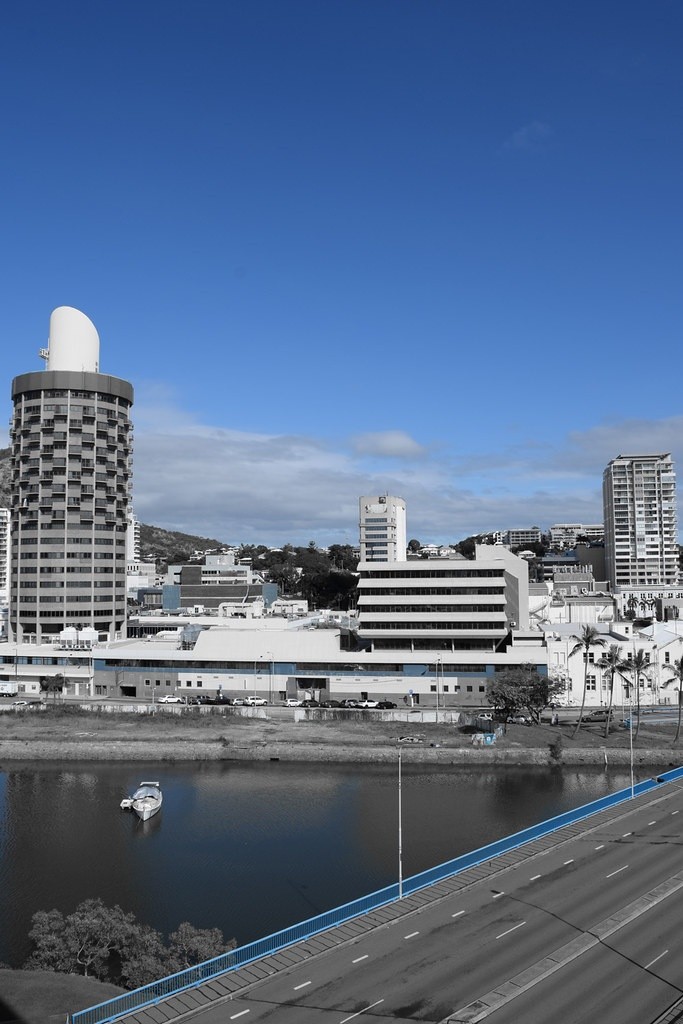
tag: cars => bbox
[478,713,493,721]
[180,695,231,705]
[340,698,359,708]
[157,695,182,704]
[26,700,44,706]
[229,698,244,706]
[301,699,322,708]
[507,714,532,725]
[12,700,27,705]
[377,701,397,709]
[283,699,302,708]
[322,699,340,708]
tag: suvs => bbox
[243,696,268,707]
[358,699,379,709]
[583,709,615,723]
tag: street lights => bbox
[437,652,445,707]
[254,656,263,707]
[436,659,440,712]
[64,653,73,688]
[267,651,275,704]
[13,648,18,680]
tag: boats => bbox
[119,781,163,821]
